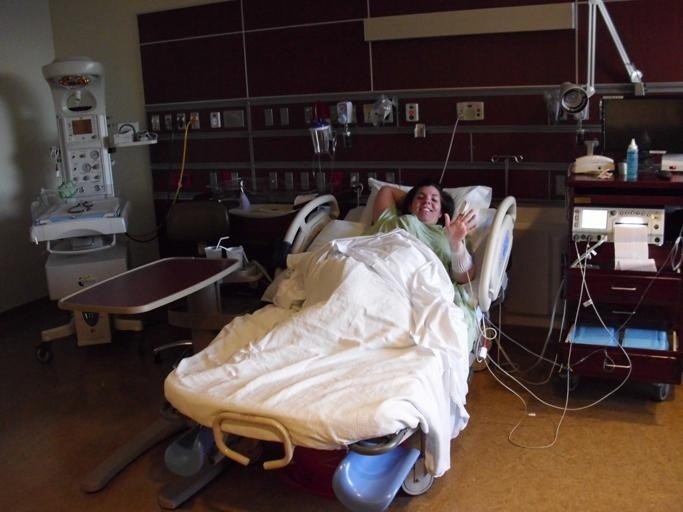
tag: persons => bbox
[358,182,478,285]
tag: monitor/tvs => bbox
[599,94,683,171]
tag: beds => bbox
[154,193,517,512]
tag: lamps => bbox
[557,1,645,113]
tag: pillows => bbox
[361,177,493,246]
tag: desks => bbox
[58,257,240,354]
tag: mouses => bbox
[656,170,672,180]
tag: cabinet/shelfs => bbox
[562,189,681,386]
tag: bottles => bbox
[626,137,639,181]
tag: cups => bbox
[310,117,329,152]
[204,246,223,259]
[226,247,243,270]
